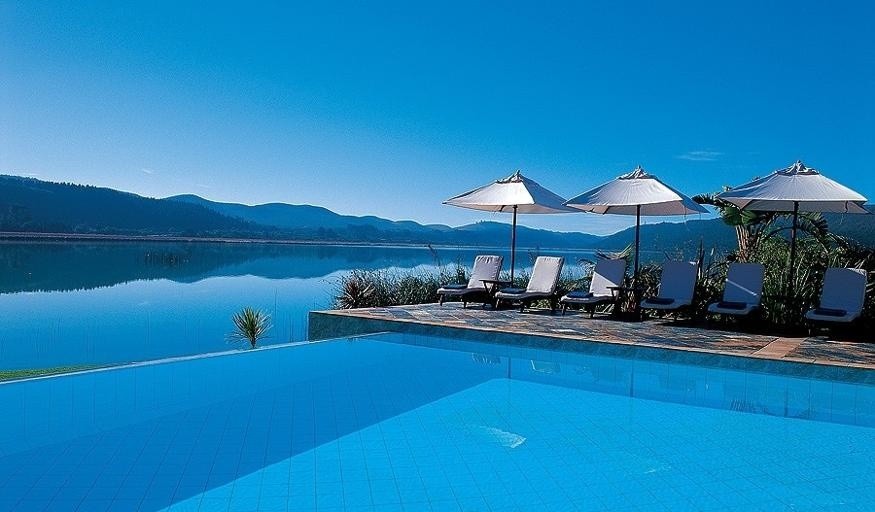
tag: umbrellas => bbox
[442,165,711,289]
[715,160,875,308]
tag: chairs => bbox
[470,352,501,368]
[803,267,868,336]
[639,261,698,324]
[436,255,506,308]
[559,258,628,319]
[494,255,565,314]
[531,359,560,376]
[703,262,766,329]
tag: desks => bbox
[608,286,644,320]
[479,279,512,309]
[767,295,805,335]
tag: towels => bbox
[500,287,527,294]
[566,290,594,298]
[646,296,675,305]
[814,307,847,317]
[717,301,747,310]
[443,284,467,289]
